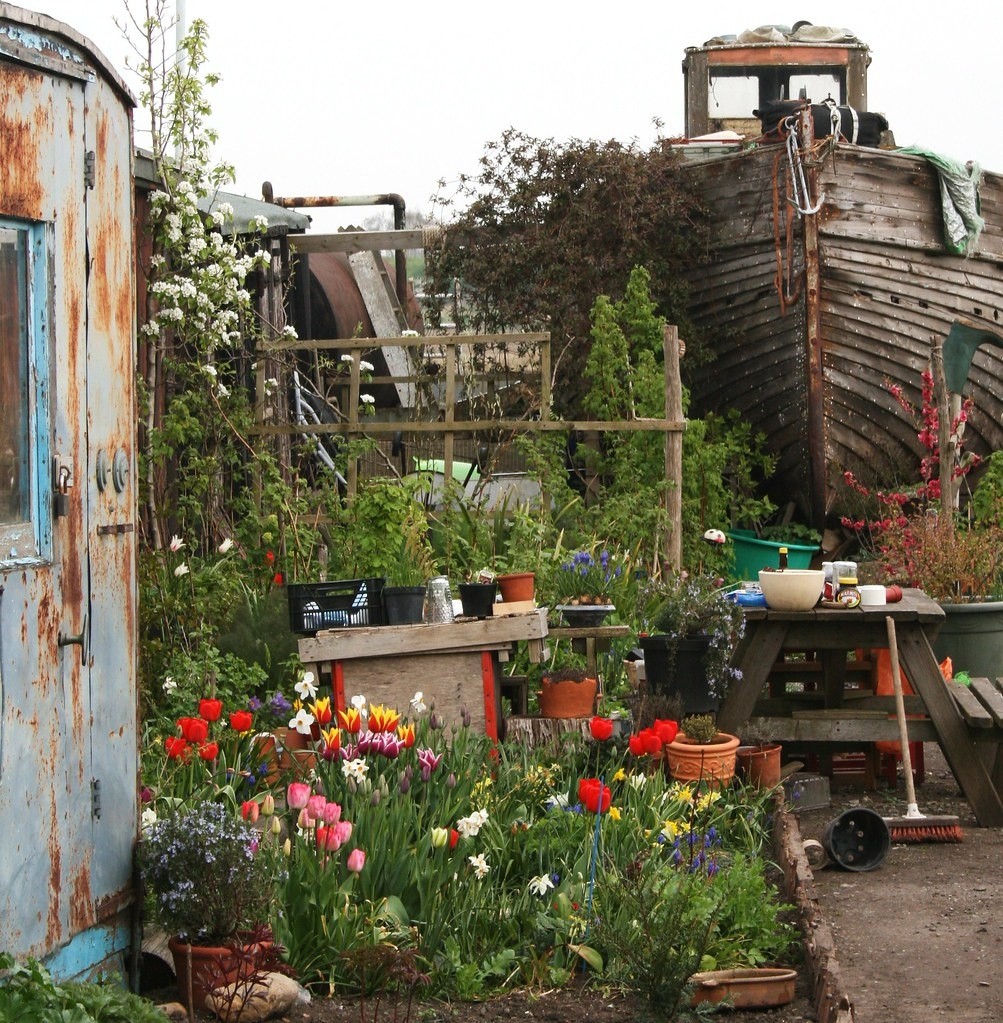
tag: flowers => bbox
[547,550,629,618]
[639,577,745,699]
[134,801,289,940]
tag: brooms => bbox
[879,616,968,844]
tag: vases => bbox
[757,569,826,611]
[168,933,274,1003]
[496,569,535,603]
[822,808,891,873]
[382,587,426,624]
[554,604,617,627]
[458,584,497,617]
[693,968,796,1002]
[639,632,716,701]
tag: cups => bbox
[861,584,886,606]
[822,561,857,602]
[424,574,455,625]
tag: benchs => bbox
[948,677,1003,727]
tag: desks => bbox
[718,589,1003,824]
[297,606,548,750]
[542,626,630,684]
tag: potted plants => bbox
[739,718,782,788]
[541,664,598,718]
[725,446,822,581]
[665,714,740,790]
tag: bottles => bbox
[779,547,790,570]
[834,578,862,608]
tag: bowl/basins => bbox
[759,569,825,611]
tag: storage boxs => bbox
[286,577,384,632]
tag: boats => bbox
[548,22,1003,562]
